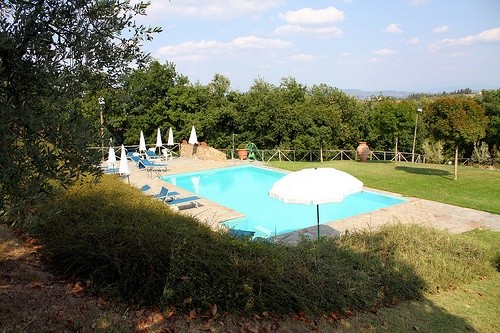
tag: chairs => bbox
[138,184,200,211]
[96,148,172,181]
[227,229,256,241]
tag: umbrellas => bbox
[139,131,146,153]
[109,138,117,168]
[157,128,162,155]
[191,176,200,193]
[168,127,174,146]
[171,178,176,185]
[119,144,130,182]
[269,168,363,239]
[189,126,198,144]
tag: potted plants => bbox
[236,144,250,160]
